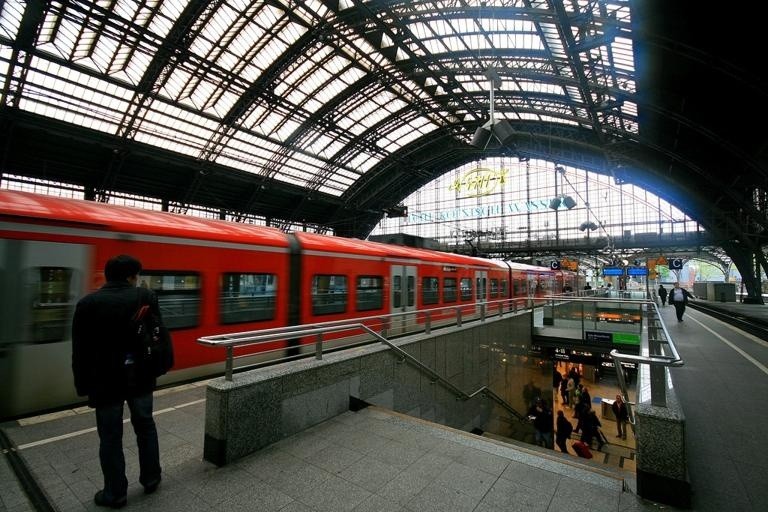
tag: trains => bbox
[0,190,576,420]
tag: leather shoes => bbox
[95,489,127,509]
[144,476,162,493]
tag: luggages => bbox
[572,442,592,459]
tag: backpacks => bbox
[125,286,174,378]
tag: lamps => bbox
[550,163,577,212]
[469,66,518,151]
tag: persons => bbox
[669,282,693,322]
[612,393,637,440]
[72,253,174,509]
[584,282,591,290]
[527,362,604,454]
[658,285,667,306]
[563,282,573,293]
